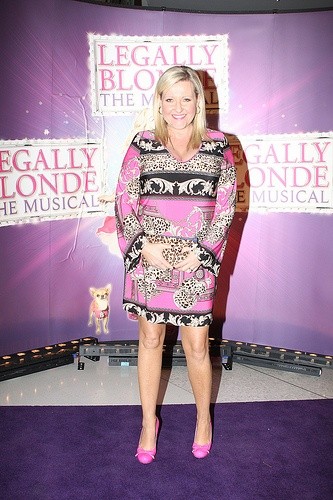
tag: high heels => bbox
[135,415,159,465]
[191,418,212,458]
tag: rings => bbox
[187,268,191,273]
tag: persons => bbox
[113,65,238,464]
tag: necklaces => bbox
[167,136,192,163]
